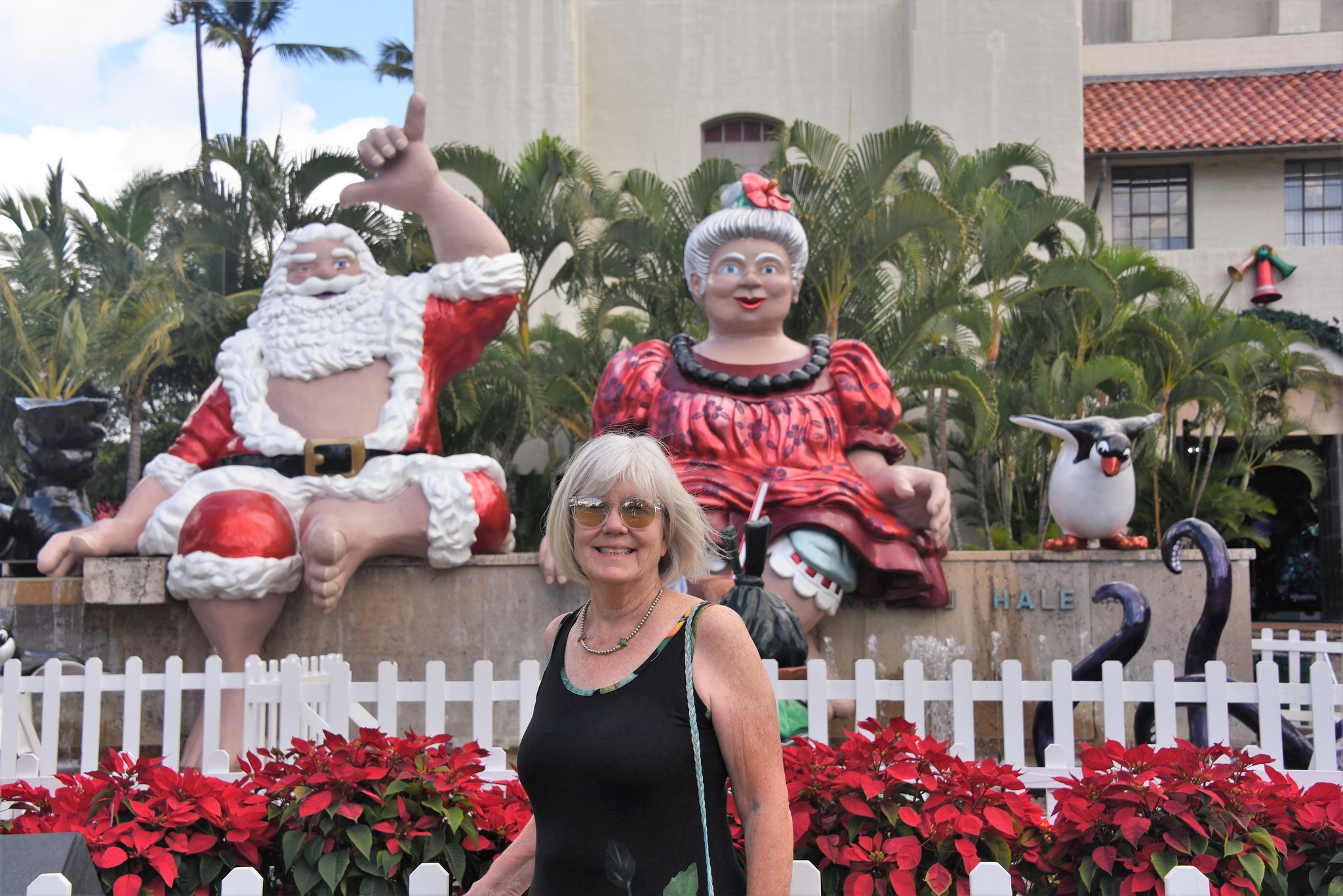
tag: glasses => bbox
[564,493,667,529]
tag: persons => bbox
[589,174,952,715]
[35,92,526,773]
[460,433,795,896]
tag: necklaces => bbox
[577,582,663,654]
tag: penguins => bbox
[1009,413,1164,550]
